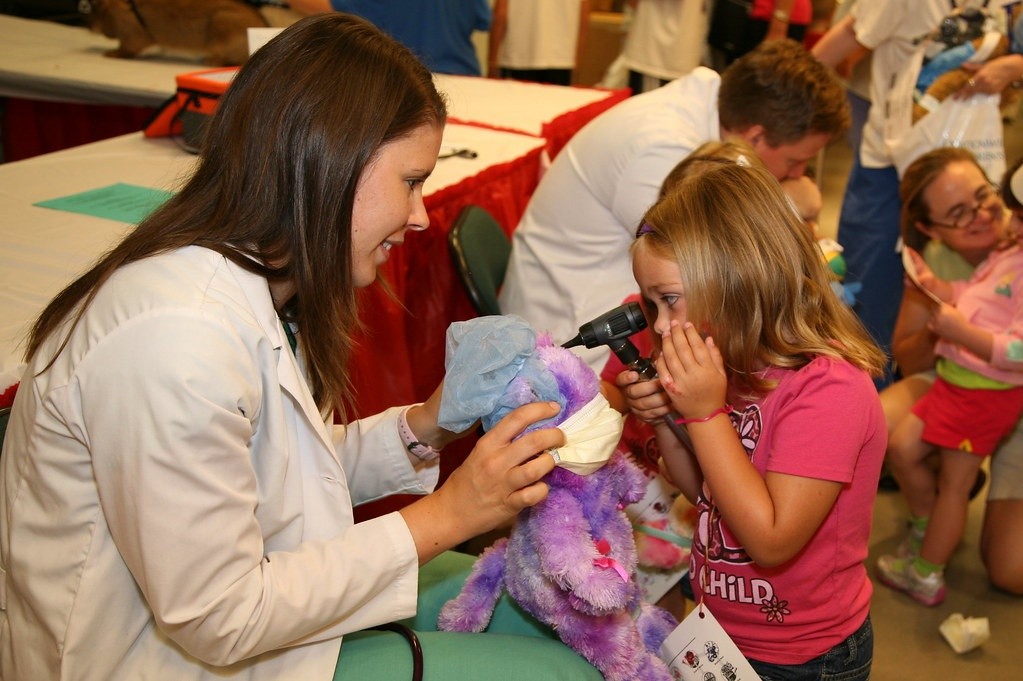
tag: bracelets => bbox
[675,404,734,424]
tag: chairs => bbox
[447,203,512,319]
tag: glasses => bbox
[928,183,1002,227]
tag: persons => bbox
[735,2,814,65]
[953,3,1023,104]
[658,142,764,203]
[879,147,1023,597]
[618,165,886,681]
[807,2,1008,394]
[609,1,716,97]
[875,159,1023,602]
[494,42,855,381]
[1,12,651,681]
[319,0,495,77]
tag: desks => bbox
[0,14,629,430]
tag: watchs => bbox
[397,403,445,461]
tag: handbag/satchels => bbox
[887,27,1009,189]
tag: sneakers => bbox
[876,531,947,607]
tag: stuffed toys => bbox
[435,334,685,681]
